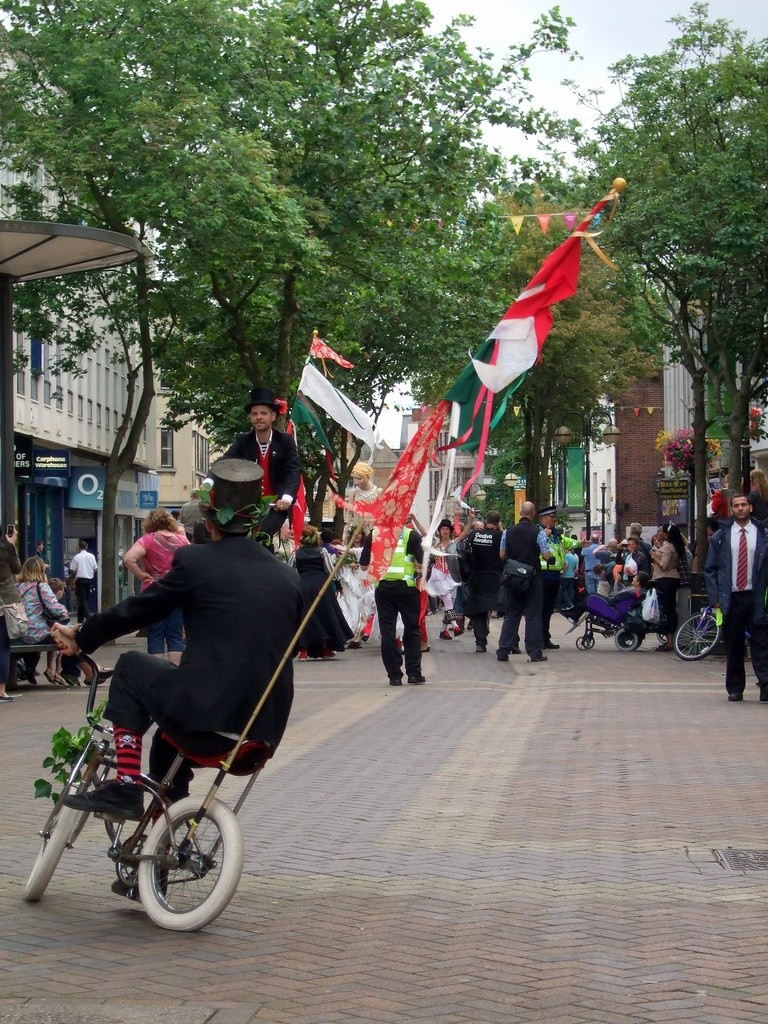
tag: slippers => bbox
[655,641,672,651]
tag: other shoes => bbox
[440,630,453,640]
[110,863,168,904]
[58,779,145,821]
[467,623,473,630]
[759,693,768,703]
[421,647,431,652]
[453,626,463,636]
[17,657,115,702]
[409,677,425,683]
[0,696,16,702]
[728,693,744,701]
[297,650,307,661]
[390,679,402,686]
[322,648,337,659]
[475,641,560,661]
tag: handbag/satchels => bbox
[641,587,660,623]
[36,581,70,628]
[500,558,537,596]
[624,553,638,576]
[2,602,29,640]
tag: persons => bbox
[51,458,305,902]
[0,463,768,703]
[201,388,301,556]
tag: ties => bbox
[736,529,747,592]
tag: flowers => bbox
[655,428,721,481]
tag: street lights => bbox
[0,216,144,535]
[450,482,487,516]
[553,411,622,539]
[600,482,607,545]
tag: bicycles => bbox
[21,627,246,933]
[672,600,722,662]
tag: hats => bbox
[298,526,320,546]
[437,519,453,534]
[199,457,269,535]
[537,505,556,517]
[244,385,281,418]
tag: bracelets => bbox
[652,555,655,559]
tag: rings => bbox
[280,505,283,508]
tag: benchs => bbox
[8,642,60,654]
